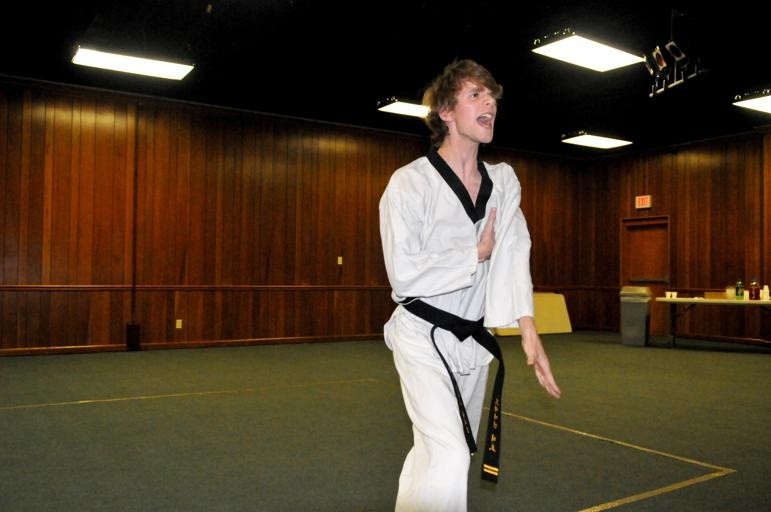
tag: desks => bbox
[655,297,771,348]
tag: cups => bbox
[743,285,770,300]
[726,288,736,300]
[664,291,678,299]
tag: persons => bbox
[378,59,562,511]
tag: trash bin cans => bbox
[619,286,653,346]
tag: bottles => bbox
[749,280,761,300]
[736,279,744,300]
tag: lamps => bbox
[530,24,647,73]
[70,40,197,81]
[732,89,771,115]
[560,129,634,150]
[644,12,716,98]
[377,96,430,118]
